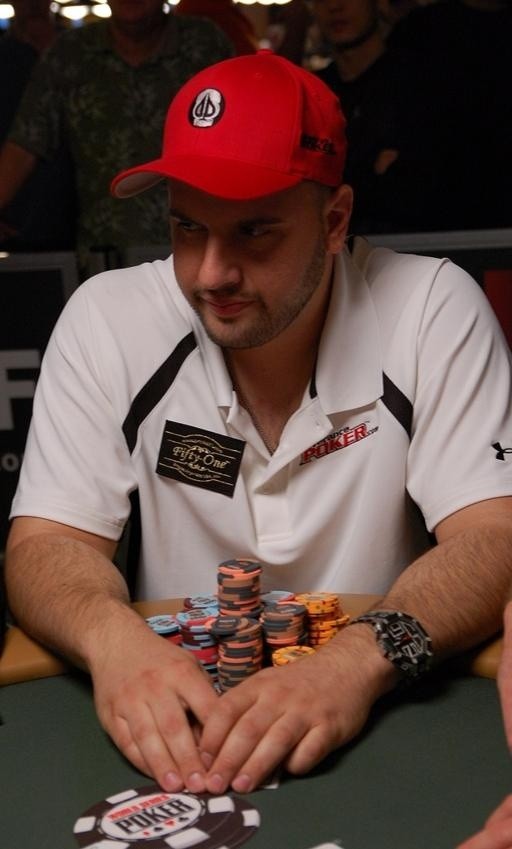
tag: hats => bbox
[110,49,347,200]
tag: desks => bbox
[0,593,512,849]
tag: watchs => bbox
[348,609,441,699]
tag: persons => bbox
[1,1,508,434]
[5,52,511,799]
